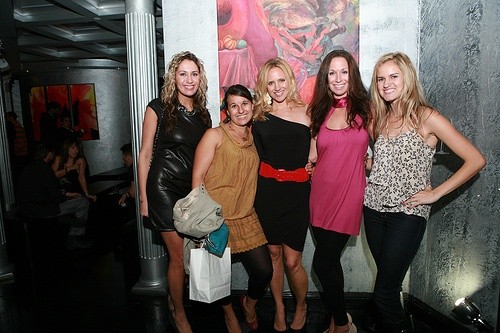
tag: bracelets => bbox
[126,192,130,198]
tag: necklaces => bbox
[227,122,249,142]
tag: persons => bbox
[42,140,96,202]
[191,84,313,333]
[306,49,375,333]
[14,141,93,249]
[250,57,368,333]
[118,143,138,208]
[6,112,27,152]
[59,113,90,149]
[39,101,61,146]
[363,51,480,333]
[137,51,213,333]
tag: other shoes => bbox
[167,293,195,333]
[322,312,357,333]
[224,312,244,333]
[240,294,259,331]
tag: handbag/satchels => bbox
[189,240,231,303]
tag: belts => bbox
[258,161,310,183]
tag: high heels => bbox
[273,304,286,333]
[288,303,311,333]
